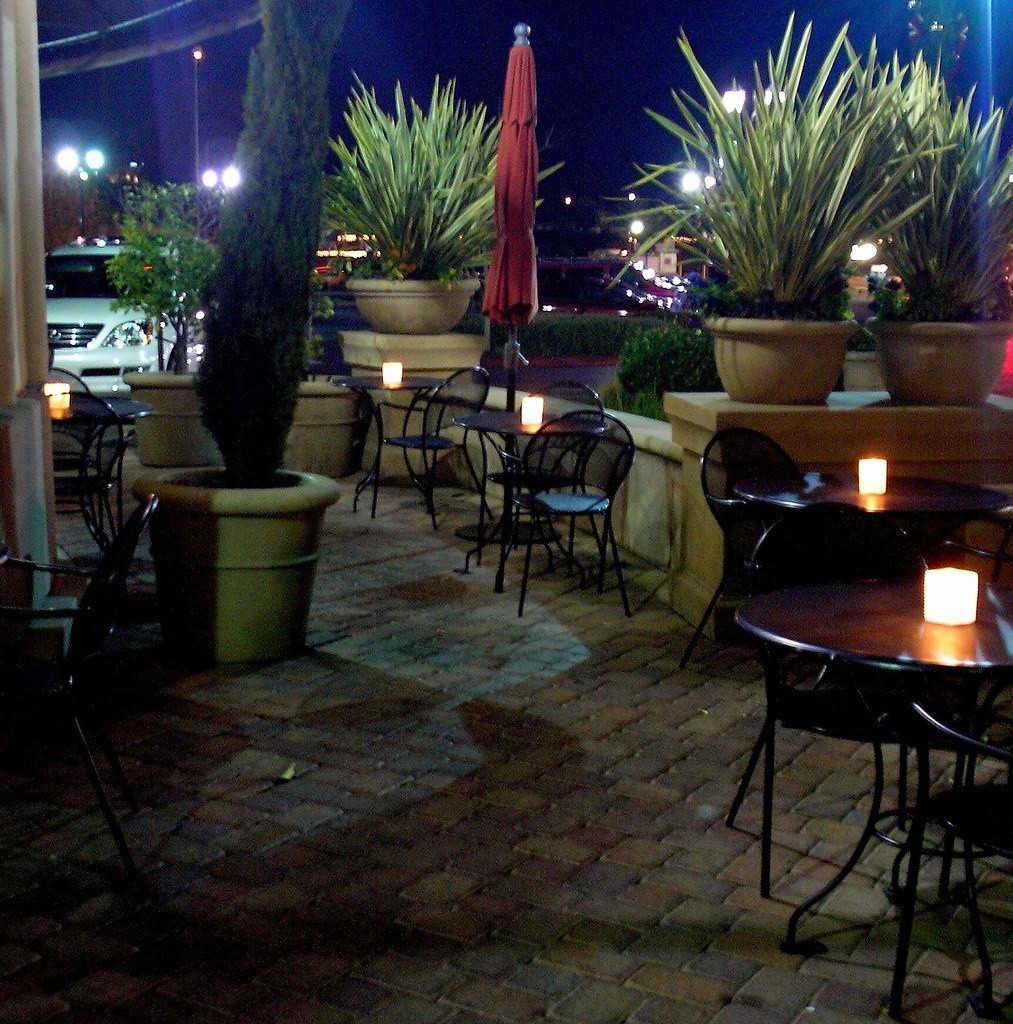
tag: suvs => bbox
[473,255,681,316]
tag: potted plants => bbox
[284,271,373,479]
[154,0,329,661]
[602,10,1013,404]
[107,183,222,466]
[319,67,564,334]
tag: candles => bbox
[44,383,69,412]
[383,363,404,384]
[923,567,979,626]
[859,459,888,493]
[522,396,543,424]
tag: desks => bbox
[735,475,1012,511]
[453,412,608,590]
[335,378,451,518]
[736,582,1013,896]
[73,397,153,539]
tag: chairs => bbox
[495,381,635,618]
[725,500,1013,1021]
[678,426,803,670]
[0,492,158,890]
[372,365,494,529]
[0,365,123,550]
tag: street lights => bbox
[55,148,106,236]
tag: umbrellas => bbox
[481,21,540,403]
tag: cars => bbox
[309,258,346,290]
[45,235,206,399]
